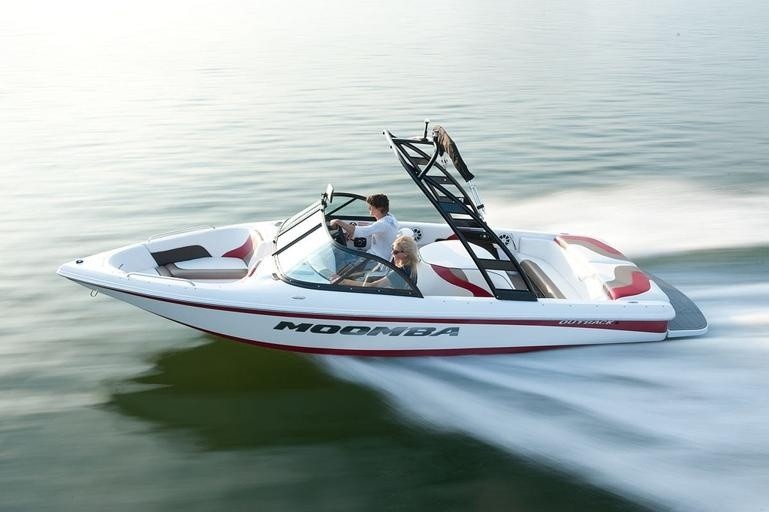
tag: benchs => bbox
[519,236,588,300]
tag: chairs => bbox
[366,227,415,282]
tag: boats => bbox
[50,114,712,359]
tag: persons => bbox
[326,234,421,291]
[330,192,397,275]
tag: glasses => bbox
[391,245,403,254]
